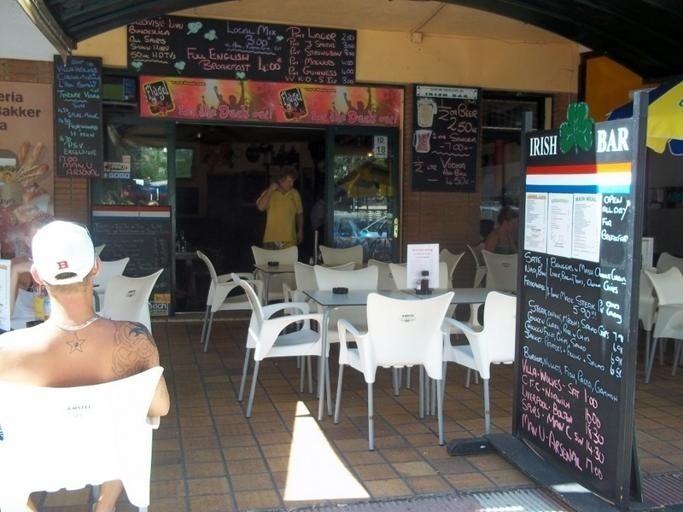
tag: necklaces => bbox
[51,313,101,332]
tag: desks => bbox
[176,250,198,311]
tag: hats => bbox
[31,220,95,286]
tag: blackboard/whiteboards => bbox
[512,192,643,506]
[127,15,357,86]
[411,83,482,193]
[53,55,103,178]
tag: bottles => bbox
[420,271,429,293]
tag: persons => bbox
[0,220,170,512]
[5,226,39,318]
[255,167,303,249]
[309,196,326,232]
[483,209,521,263]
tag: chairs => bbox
[1,366,164,511]
[0,243,164,330]
[196,243,519,446]
[637,253,683,384]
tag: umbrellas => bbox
[601,80,682,156]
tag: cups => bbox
[33,284,47,321]
[413,130,432,153]
[417,99,438,127]
